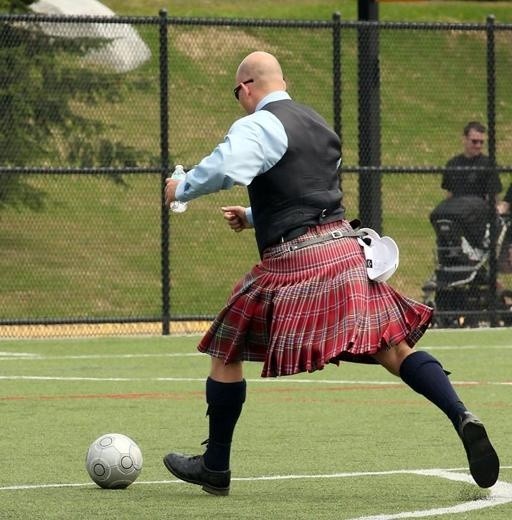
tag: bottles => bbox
[169,163,189,214]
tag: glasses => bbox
[233,77,254,101]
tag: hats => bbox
[354,224,401,285]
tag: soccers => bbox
[86,435,143,489]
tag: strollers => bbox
[422,195,512,328]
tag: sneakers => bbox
[162,449,232,497]
[456,410,500,491]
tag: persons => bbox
[162,51,499,496]
[441,121,512,273]
